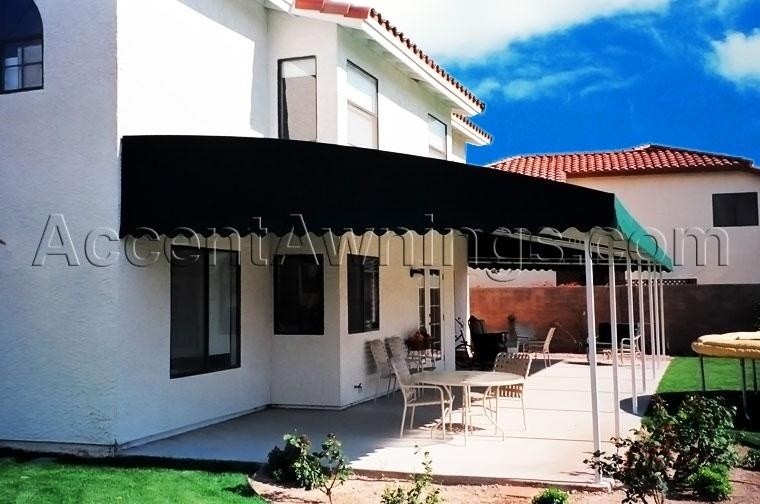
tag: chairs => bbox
[522,328,556,369]
[390,357,452,440]
[461,352,532,433]
[369,336,437,403]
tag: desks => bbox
[414,371,525,448]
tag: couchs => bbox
[587,322,642,367]
[467,315,508,362]
